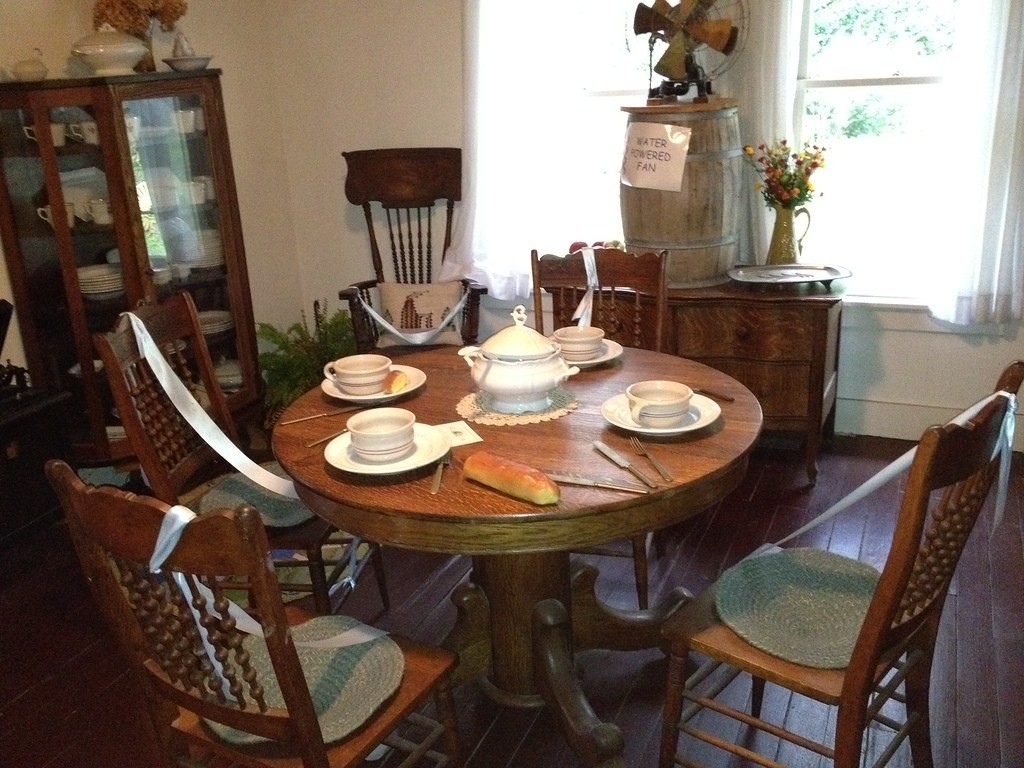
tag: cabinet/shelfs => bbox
[545,282,846,483]
[0,384,78,549]
[0,68,264,471]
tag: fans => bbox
[634,0,751,103]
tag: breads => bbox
[383,370,408,394]
[462,450,561,505]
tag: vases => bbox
[764,204,811,267]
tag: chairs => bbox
[659,360,1023,768]
[46,459,463,768]
[531,248,671,610]
[99,289,391,623]
[337,147,488,349]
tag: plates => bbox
[548,336,624,369]
[320,364,427,404]
[69,172,226,376]
[324,422,451,476]
[726,262,852,283]
[600,393,721,437]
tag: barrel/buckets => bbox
[620,98,743,289]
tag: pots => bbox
[458,304,580,415]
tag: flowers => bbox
[745,139,827,212]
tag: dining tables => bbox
[267,338,764,750]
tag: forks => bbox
[628,436,673,482]
[429,449,451,495]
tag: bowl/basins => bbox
[162,55,212,72]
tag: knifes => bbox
[592,440,659,488]
[546,473,649,495]
[280,405,366,426]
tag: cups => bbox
[324,354,392,396]
[84,198,115,225]
[23,106,206,147]
[346,406,416,462]
[553,325,605,361]
[37,201,75,231]
[625,380,694,429]
